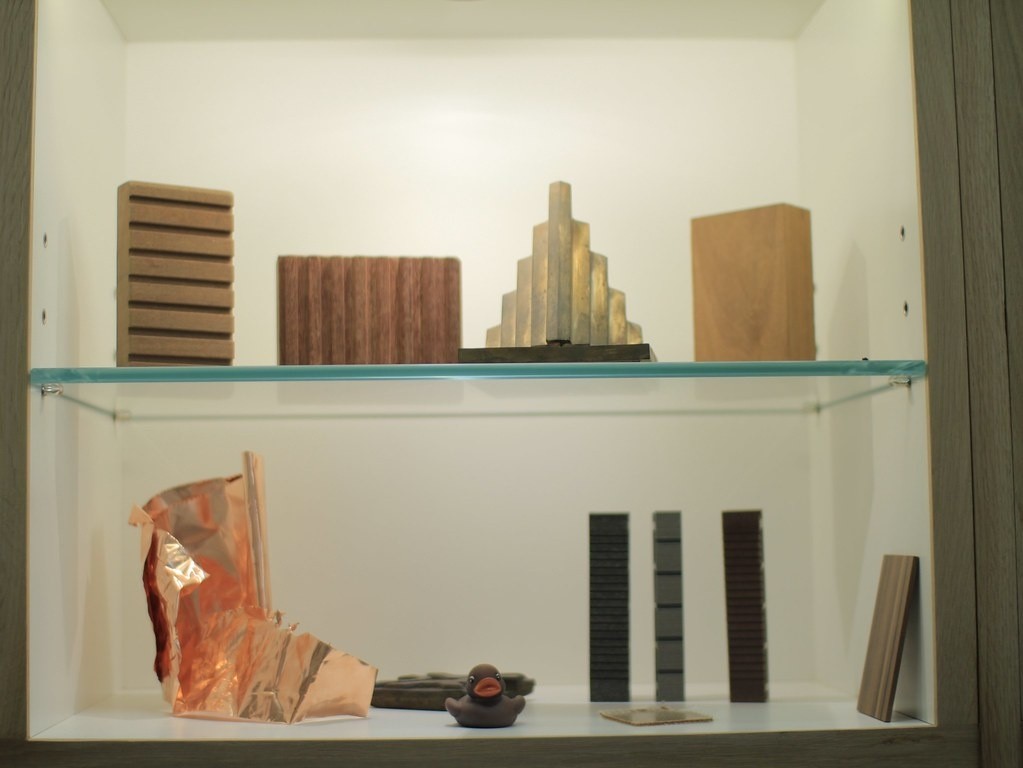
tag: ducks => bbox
[443,662,526,729]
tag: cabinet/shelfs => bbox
[0,1,1023,768]
